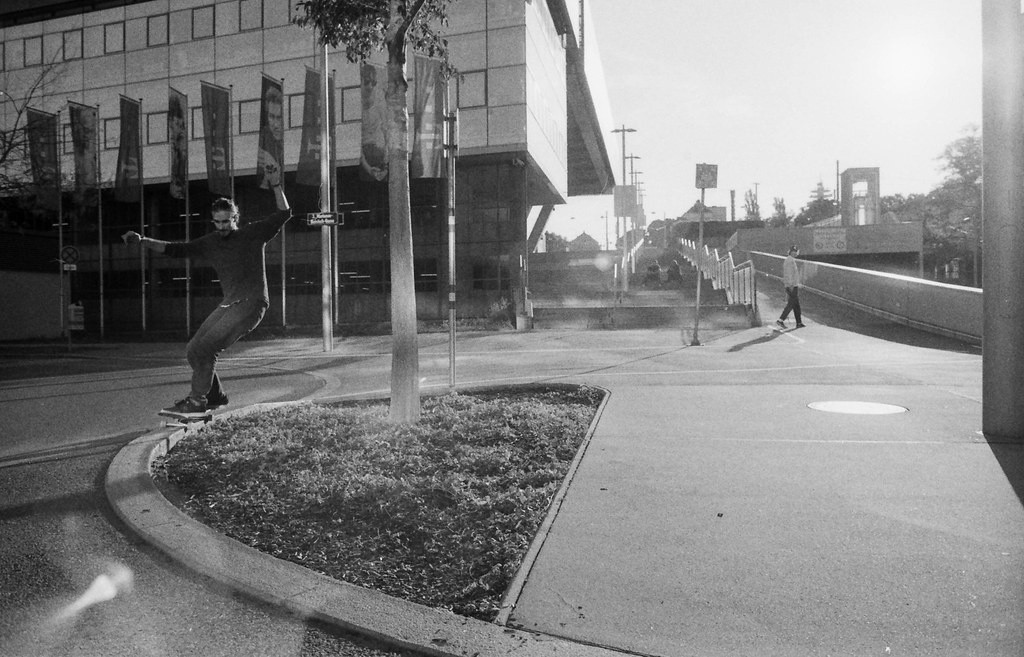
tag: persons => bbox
[666,260,681,287]
[776,245,806,329]
[641,260,661,289]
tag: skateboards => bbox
[157,401,226,430]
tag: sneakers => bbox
[796,324,805,328]
[206,394,229,408]
[777,320,788,329]
[160,396,207,418]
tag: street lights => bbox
[611,124,648,295]
[600,210,609,250]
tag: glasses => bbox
[214,215,235,223]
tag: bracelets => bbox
[138,235,145,246]
[120,164,293,419]
[270,182,280,187]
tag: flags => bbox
[24,55,442,208]
